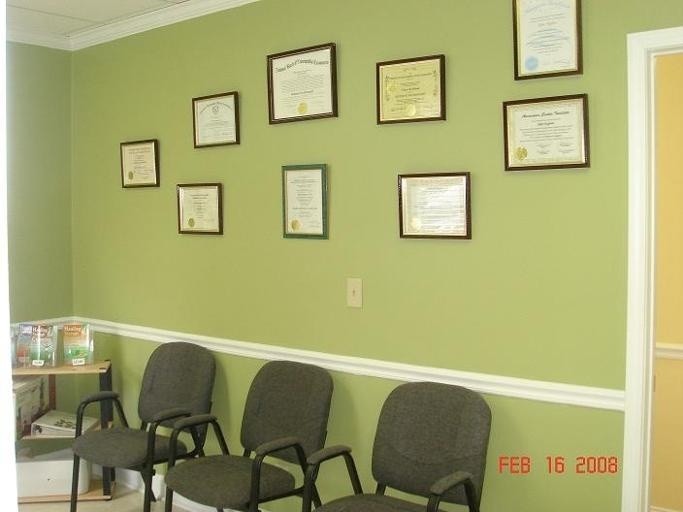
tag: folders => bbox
[30,409,99,439]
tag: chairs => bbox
[165,359,333,512]
[70,343,215,512]
[303,382,492,512]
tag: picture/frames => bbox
[191,91,240,148]
[280,164,328,240]
[266,42,337,124]
[119,138,159,188]
[502,94,589,170]
[397,172,471,240]
[176,183,223,235]
[376,54,446,124]
[511,0,582,81]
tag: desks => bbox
[12,359,116,502]
